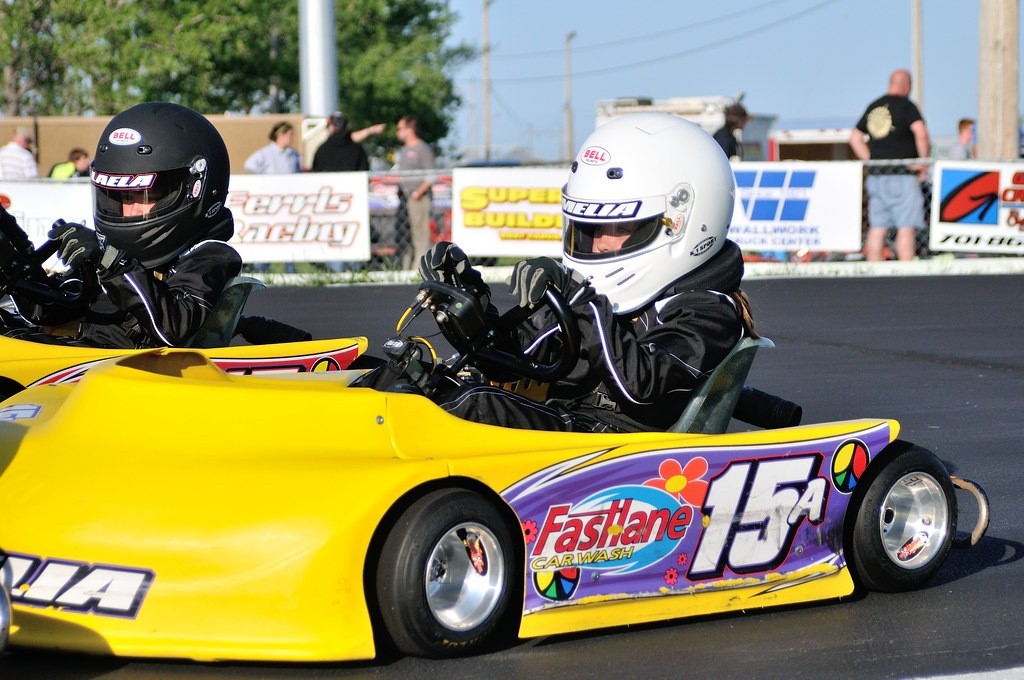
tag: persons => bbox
[244,123,301,274]
[850,70,929,262]
[0,127,38,177]
[713,105,746,160]
[310,111,387,272]
[390,116,435,270]
[48,148,92,177]
[419,112,755,433]
[939,119,975,158]
[0,101,242,348]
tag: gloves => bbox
[505,256,591,307]
[48,223,120,275]
[418,241,492,317]
[0,202,34,295]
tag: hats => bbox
[327,111,349,129]
[725,105,753,122]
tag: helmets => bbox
[561,112,736,314]
[90,102,235,271]
